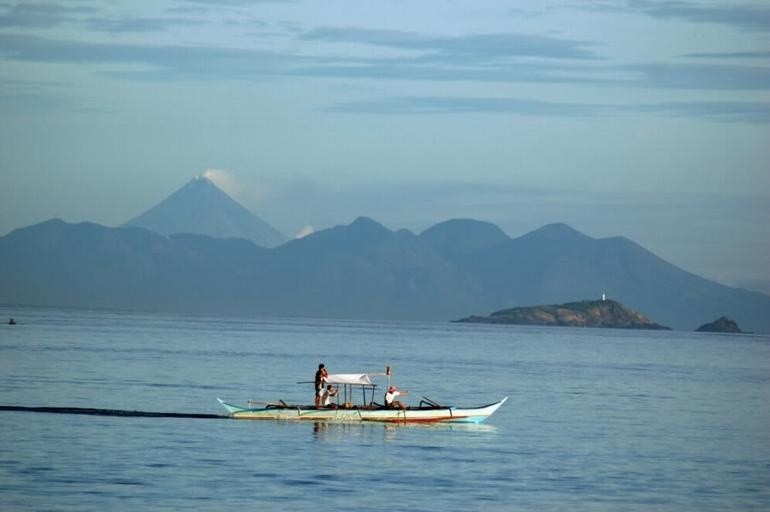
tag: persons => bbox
[384,386,409,410]
[314,363,329,410]
[322,385,339,409]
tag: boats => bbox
[214,393,513,422]
